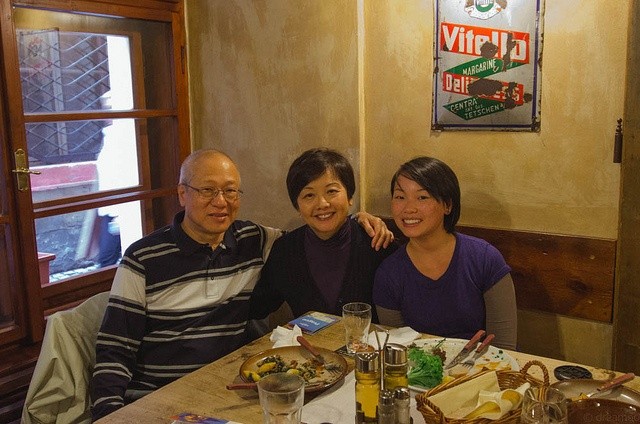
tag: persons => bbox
[88,149,394,424]
[374,156,517,351]
[248,150,399,336]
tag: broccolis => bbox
[407,353,443,387]
[407,349,423,361]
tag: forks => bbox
[459,334,494,373]
[297,336,343,380]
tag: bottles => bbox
[395,387,410,424]
[377,389,396,424]
[355,352,381,424]
[383,343,408,394]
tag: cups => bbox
[257,373,305,424]
[342,301,372,356]
[520,386,568,424]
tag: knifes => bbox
[445,330,486,371]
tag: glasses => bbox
[178,182,244,200]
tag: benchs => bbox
[362,214,617,369]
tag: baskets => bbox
[415,360,549,423]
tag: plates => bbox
[400,337,520,393]
[239,346,348,393]
[551,378,640,407]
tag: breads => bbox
[463,400,501,419]
[500,390,523,410]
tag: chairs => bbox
[19,290,111,423]
